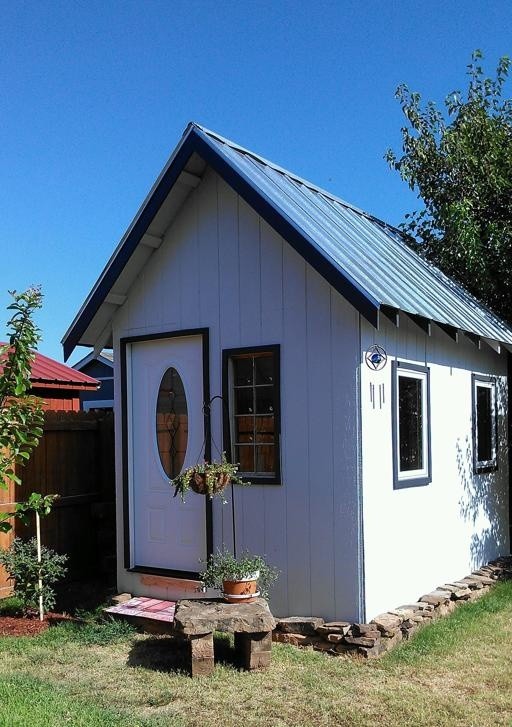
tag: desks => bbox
[174,598,277,677]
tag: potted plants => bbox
[193,545,280,603]
[171,449,251,505]
